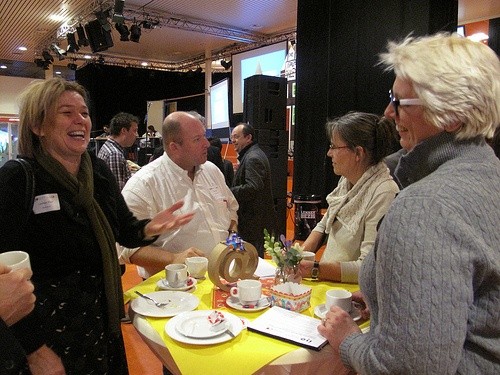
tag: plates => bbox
[176,311,229,338]
[131,291,199,317]
[314,303,362,321]
[226,294,271,311]
[157,277,197,290]
[165,310,244,345]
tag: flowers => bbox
[263,228,304,265]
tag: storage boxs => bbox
[269,282,312,313]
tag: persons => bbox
[0,261,37,340]
[96,124,110,138]
[317,29,500,375]
[229,123,275,259]
[283,112,400,283]
[98,112,139,191]
[115,111,240,282]
[140,125,163,148]
[0,77,196,375]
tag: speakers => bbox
[294,0,460,210]
[243,74,287,128]
[251,128,289,242]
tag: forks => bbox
[134,291,169,308]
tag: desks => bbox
[90,137,160,156]
[121,259,370,375]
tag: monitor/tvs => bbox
[210,77,231,129]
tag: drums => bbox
[149,137,159,148]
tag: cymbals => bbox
[136,137,144,139]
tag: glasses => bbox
[329,145,351,154]
[389,87,421,118]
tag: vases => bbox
[275,264,303,285]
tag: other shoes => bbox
[121,314,132,324]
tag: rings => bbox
[321,317,330,326]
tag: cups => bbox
[301,251,315,262]
[230,280,262,306]
[185,257,208,278]
[325,288,352,312]
[0,251,31,273]
[165,264,188,288]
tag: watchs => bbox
[311,261,319,279]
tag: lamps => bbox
[33,0,232,71]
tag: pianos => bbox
[90,137,108,156]
